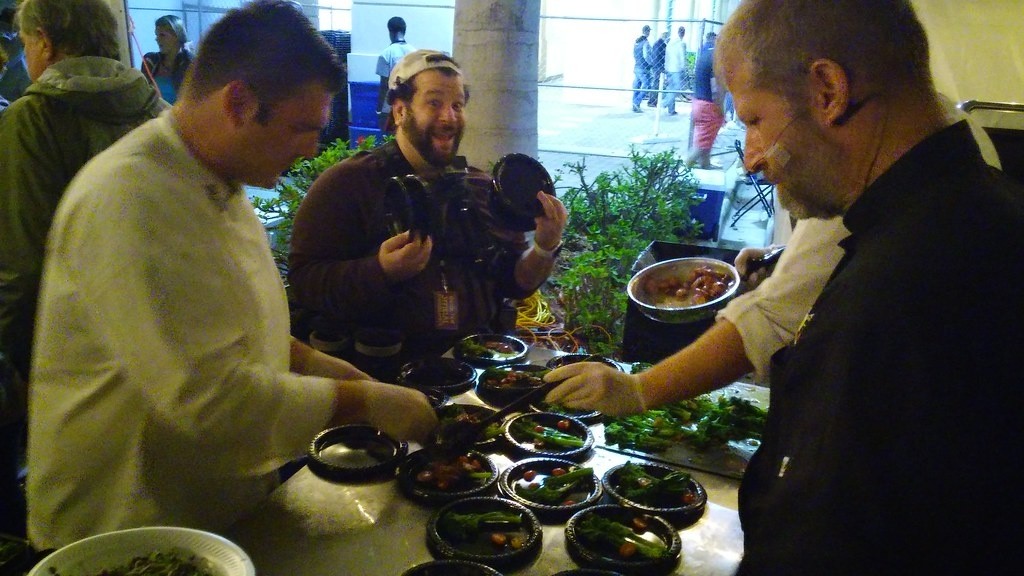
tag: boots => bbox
[698,152,723,169]
[681,148,702,172]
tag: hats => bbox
[387,49,462,92]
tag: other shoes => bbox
[633,106,643,113]
[667,111,678,116]
[648,100,656,106]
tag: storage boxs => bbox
[346,79,383,149]
[316,29,351,143]
[663,167,725,240]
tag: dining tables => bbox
[223,346,770,576]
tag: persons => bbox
[0,0,194,382]
[682,33,734,170]
[374,16,417,128]
[632,24,687,115]
[287,50,567,358]
[26,0,439,550]
[543,95,1000,417]
[713,0,1024,576]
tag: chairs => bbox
[731,139,778,229]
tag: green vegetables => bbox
[431,337,765,560]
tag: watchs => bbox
[534,237,563,259]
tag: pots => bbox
[627,245,787,325]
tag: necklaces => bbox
[390,39,406,44]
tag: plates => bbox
[384,174,439,244]
[400,356,477,391]
[503,411,591,457]
[486,154,556,232]
[497,457,603,511]
[529,403,602,419]
[600,464,707,513]
[426,495,542,565]
[406,385,448,411]
[547,355,623,371]
[551,568,621,576]
[400,559,504,576]
[308,423,408,473]
[455,332,529,363]
[565,504,681,568]
[434,405,504,448]
[396,448,499,497]
[479,364,552,395]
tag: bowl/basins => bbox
[26,525,255,576]
